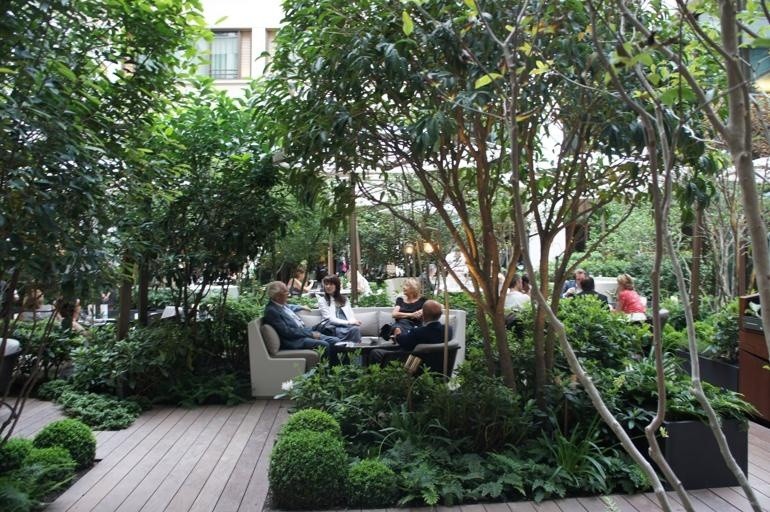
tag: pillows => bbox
[439,314,456,332]
[301,315,321,329]
[260,324,280,353]
[379,311,395,332]
[354,311,378,337]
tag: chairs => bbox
[0,338,22,396]
[404,341,461,384]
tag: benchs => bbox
[594,277,632,301]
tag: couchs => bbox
[248,305,467,399]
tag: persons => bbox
[379,276,428,344]
[368,299,453,367]
[559,268,589,298]
[318,275,362,366]
[19,289,61,322]
[573,276,610,312]
[346,270,373,304]
[522,273,532,297]
[609,273,646,315]
[284,268,315,298]
[264,279,341,366]
[419,263,442,296]
[53,296,89,336]
[503,273,530,309]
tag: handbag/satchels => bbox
[381,323,392,341]
[312,318,336,336]
